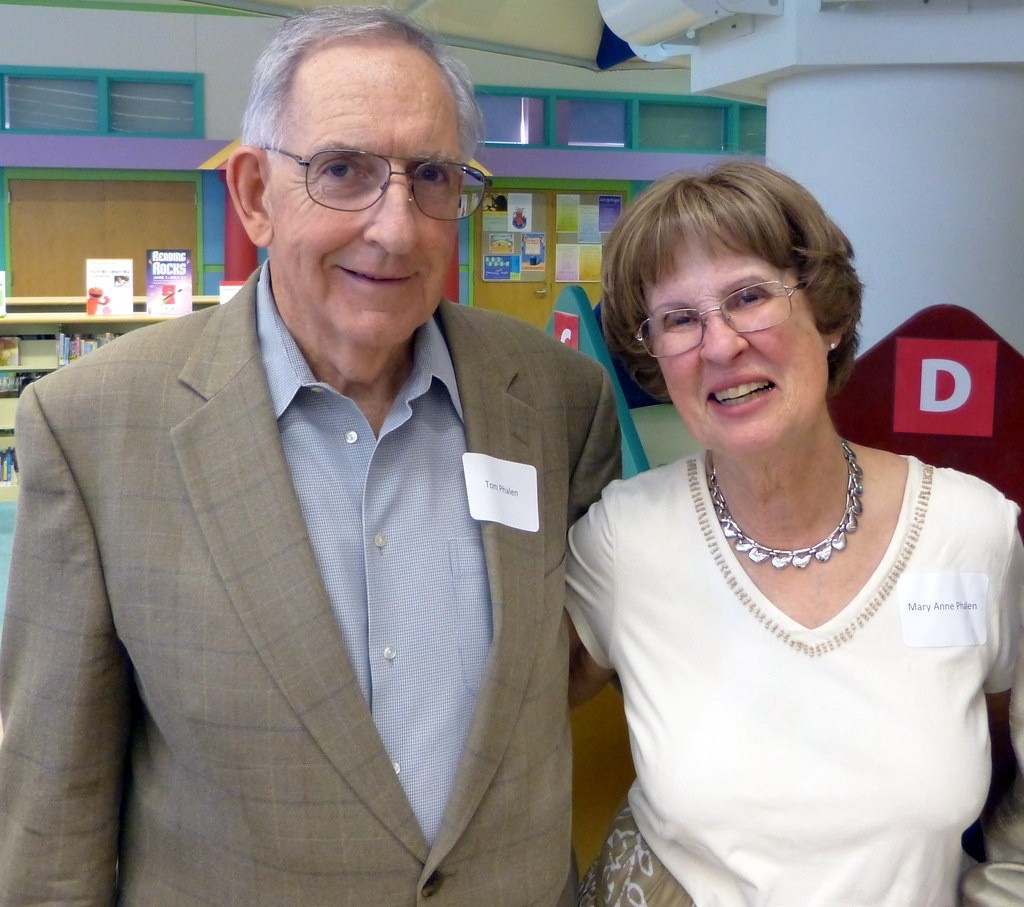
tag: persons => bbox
[552,153,1024,906]
[0,2,626,906]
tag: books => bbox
[0,336,22,366]
[0,371,46,400]
[84,258,135,316]
[58,330,119,366]
[145,248,193,318]
[0,450,12,482]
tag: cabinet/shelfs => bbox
[0,296,220,506]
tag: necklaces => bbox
[699,435,862,567]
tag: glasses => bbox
[631,277,810,357]
[257,143,492,221]
